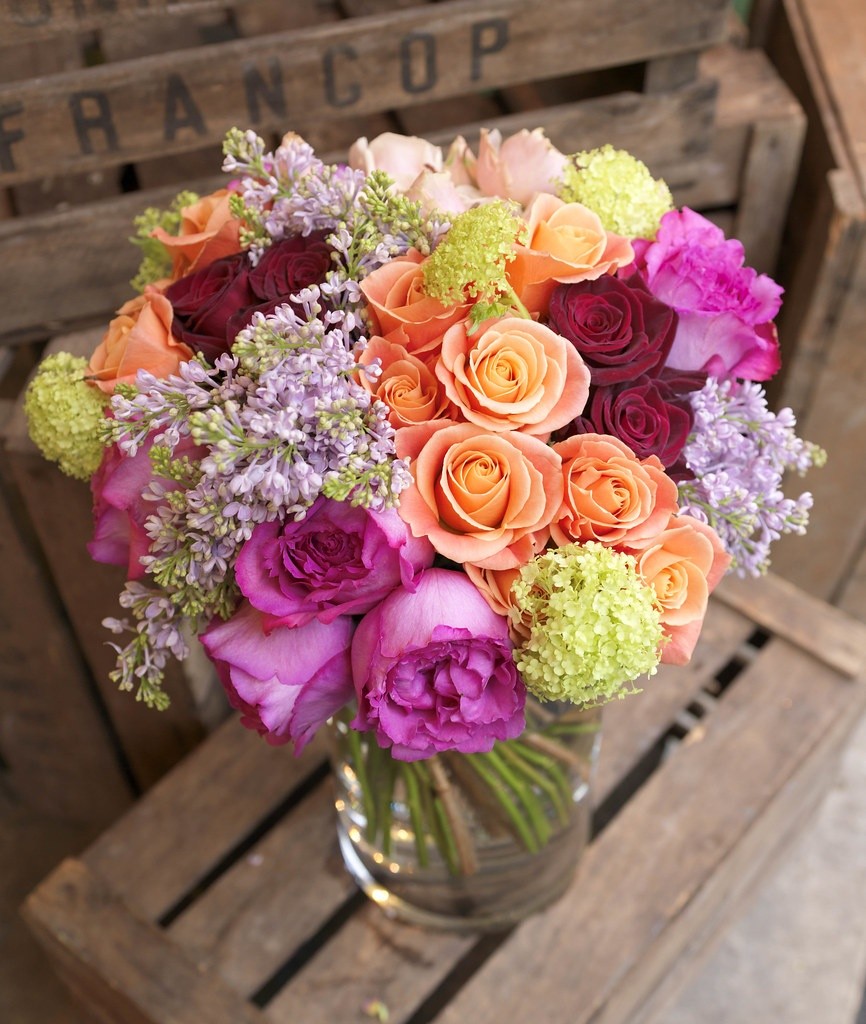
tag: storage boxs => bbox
[1,486,135,823]
[0,0,739,346]
[20,590,865,1024]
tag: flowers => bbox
[23,122,827,759]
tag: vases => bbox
[322,700,605,937]
